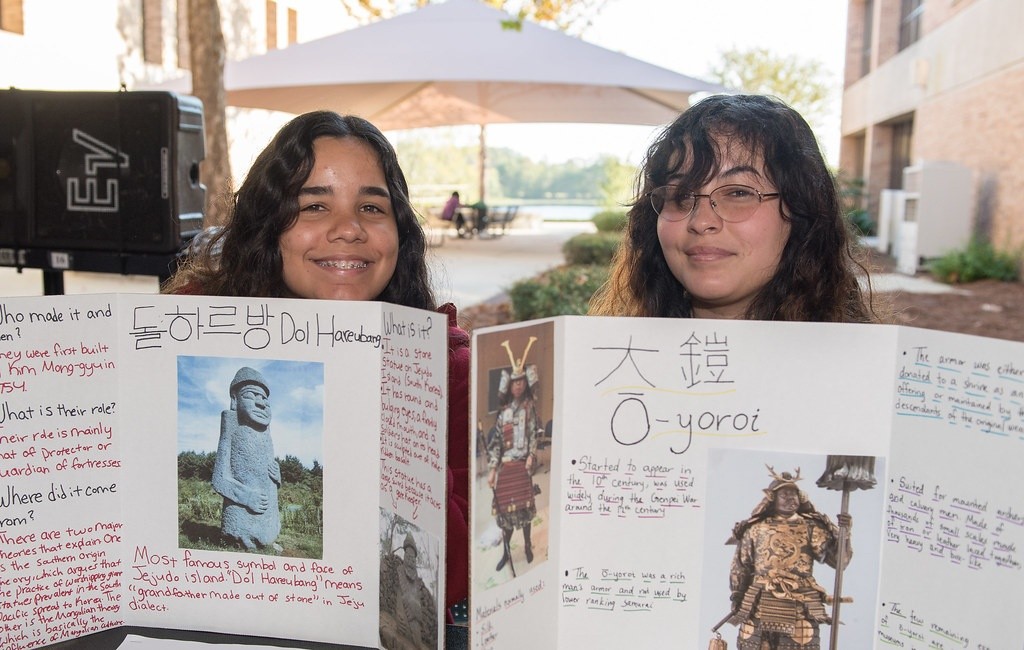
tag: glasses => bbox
[645,184,781,223]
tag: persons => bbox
[731,478,852,650]
[440,191,475,240]
[485,364,539,570]
[587,96,884,324]
[160,111,470,625]
[396,531,424,650]
[212,367,281,551]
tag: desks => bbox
[463,205,501,233]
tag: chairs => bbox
[436,204,520,240]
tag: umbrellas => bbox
[124,0,743,240]
